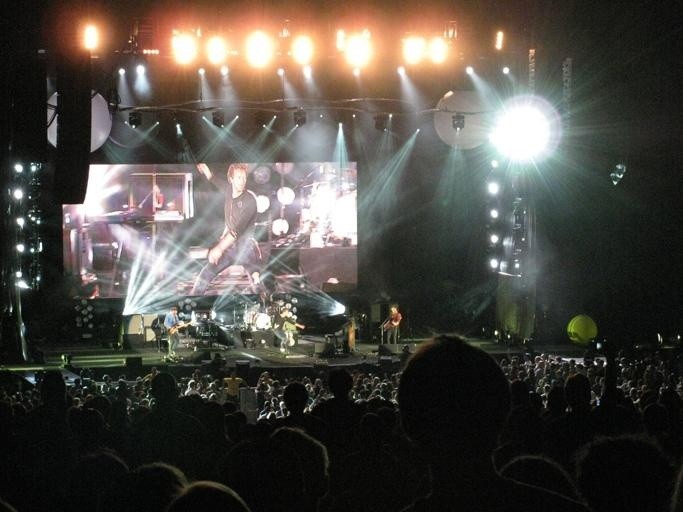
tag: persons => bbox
[0,336,683,512]
[188,164,264,297]
[163,307,182,360]
[266,306,305,351]
[383,303,403,344]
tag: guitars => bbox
[168,317,191,335]
[384,315,393,329]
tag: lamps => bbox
[129,106,465,131]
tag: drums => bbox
[252,313,271,330]
